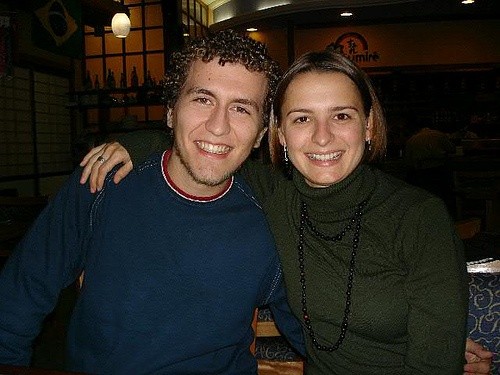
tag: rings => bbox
[97,156,106,163]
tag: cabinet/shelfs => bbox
[66,84,170,171]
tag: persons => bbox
[79,49,472,375]
[0,30,495,375]
[404,119,457,195]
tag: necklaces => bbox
[299,201,365,352]
